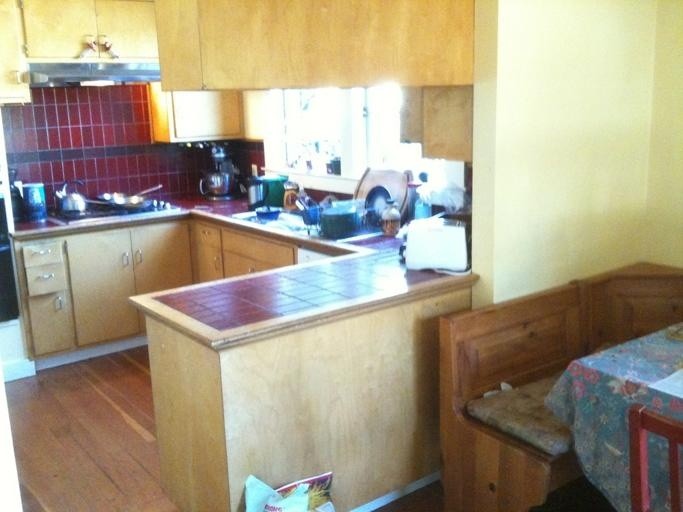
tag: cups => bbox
[304,205,320,226]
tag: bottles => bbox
[381,199,400,235]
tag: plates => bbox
[362,186,391,225]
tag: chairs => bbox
[626,402,683,512]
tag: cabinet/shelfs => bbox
[63,213,194,350]
[21,0,159,63]
[22,241,74,362]
[190,212,223,284]
[221,221,295,279]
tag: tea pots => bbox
[55,181,88,214]
[245,177,269,211]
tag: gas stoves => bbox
[52,198,180,224]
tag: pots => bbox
[96,184,163,201]
[320,209,363,237]
[87,196,155,207]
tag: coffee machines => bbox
[199,146,240,201]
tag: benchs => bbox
[438,262,683,512]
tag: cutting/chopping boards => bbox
[351,167,414,228]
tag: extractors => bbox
[30,63,161,85]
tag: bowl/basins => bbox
[254,206,278,221]
